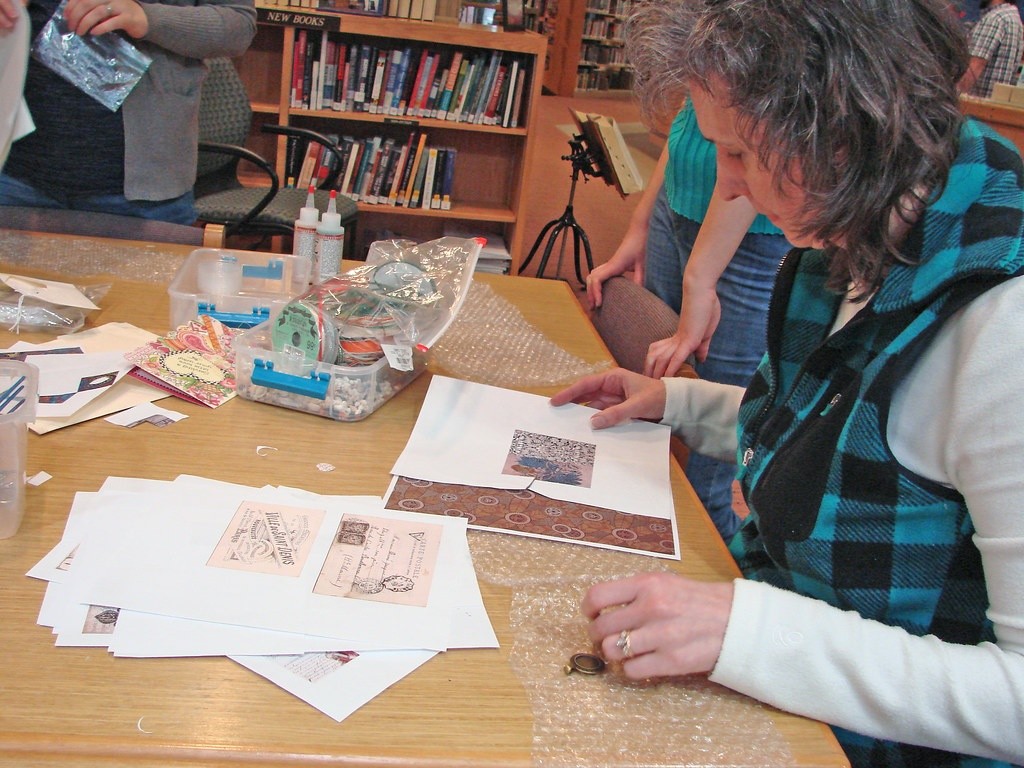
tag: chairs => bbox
[592,278,700,464]
[193,55,361,260]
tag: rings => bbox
[105,3,112,17]
[616,629,634,659]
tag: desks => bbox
[0,229,852,768]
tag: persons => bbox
[0,0,257,225]
[551,0,1023,768]
[586,94,792,545]
[954,0,1024,98]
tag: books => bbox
[344,223,512,274]
[288,133,455,210]
[291,28,525,127]
[264,0,437,21]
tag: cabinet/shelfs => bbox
[465,0,637,98]
[235,5,548,276]
